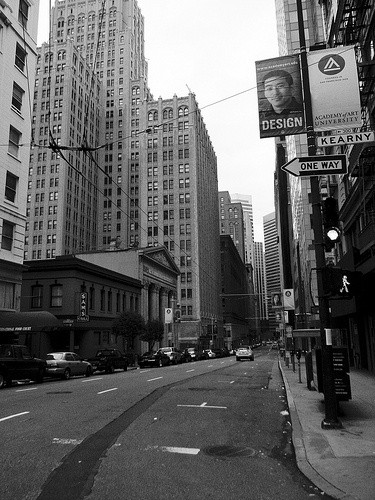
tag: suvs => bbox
[0,344,46,388]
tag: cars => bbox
[44,351,92,380]
[235,344,254,361]
[93,349,129,374]
[136,347,237,368]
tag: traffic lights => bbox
[329,267,357,297]
[321,197,344,244]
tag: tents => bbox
[0,311,62,358]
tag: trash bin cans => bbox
[322,346,352,402]
[306,351,313,380]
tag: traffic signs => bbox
[280,155,348,176]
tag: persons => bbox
[127,347,135,367]
[259,70,306,137]
[273,294,281,306]
[168,330,173,346]
